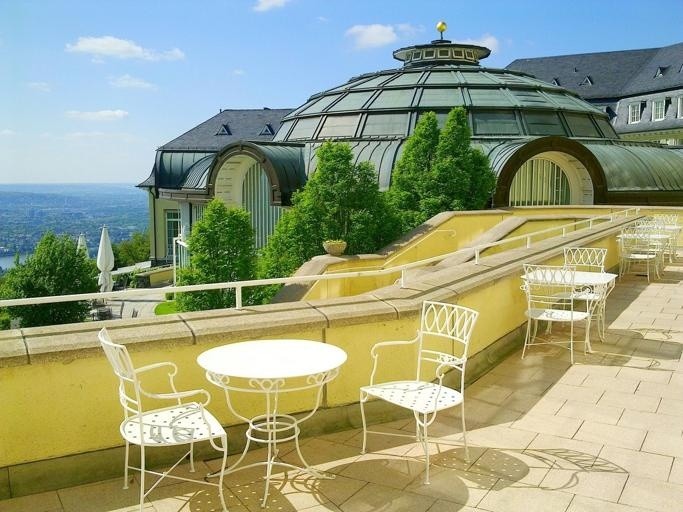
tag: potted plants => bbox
[321,239,347,256]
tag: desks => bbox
[195,338,348,509]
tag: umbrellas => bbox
[75,232,89,257]
[97,227,115,292]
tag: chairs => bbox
[519,213,682,366]
[357,299,479,486]
[97,326,229,511]
